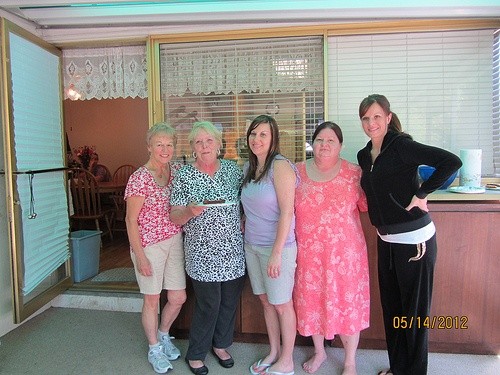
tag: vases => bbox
[81,160,90,172]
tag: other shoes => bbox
[211,346,234,368]
[185,357,208,375]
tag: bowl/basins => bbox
[418,166,458,190]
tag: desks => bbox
[68,180,128,239]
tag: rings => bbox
[273,269,278,271]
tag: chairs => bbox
[67,164,137,247]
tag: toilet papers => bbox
[458,148,482,194]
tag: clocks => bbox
[265,102,280,115]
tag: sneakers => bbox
[148,345,174,373]
[157,334,181,361]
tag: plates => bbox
[197,202,237,207]
[450,188,485,193]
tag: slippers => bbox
[259,368,295,375]
[250,359,273,374]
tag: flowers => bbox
[74,144,99,167]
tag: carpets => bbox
[92,267,137,281]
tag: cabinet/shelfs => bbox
[331,200,500,354]
[159,215,327,346]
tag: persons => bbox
[124,122,188,374]
[239,114,301,375]
[169,121,244,375]
[294,122,370,375]
[357,94,464,375]
